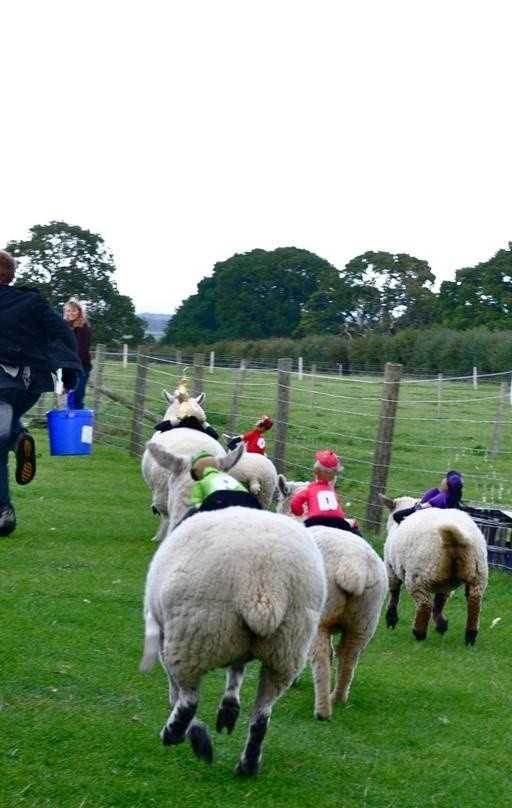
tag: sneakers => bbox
[1,510,16,536]
[14,430,35,486]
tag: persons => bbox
[393,469,464,526]
[170,449,263,532]
[291,449,364,540]
[60,301,92,411]
[228,413,274,456]
[155,392,220,440]
[0,250,84,537]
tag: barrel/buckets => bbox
[46,389,96,455]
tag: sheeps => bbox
[375,490,491,648]
[144,437,330,780]
[274,472,391,723]
[223,431,277,511]
[143,389,226,543]
